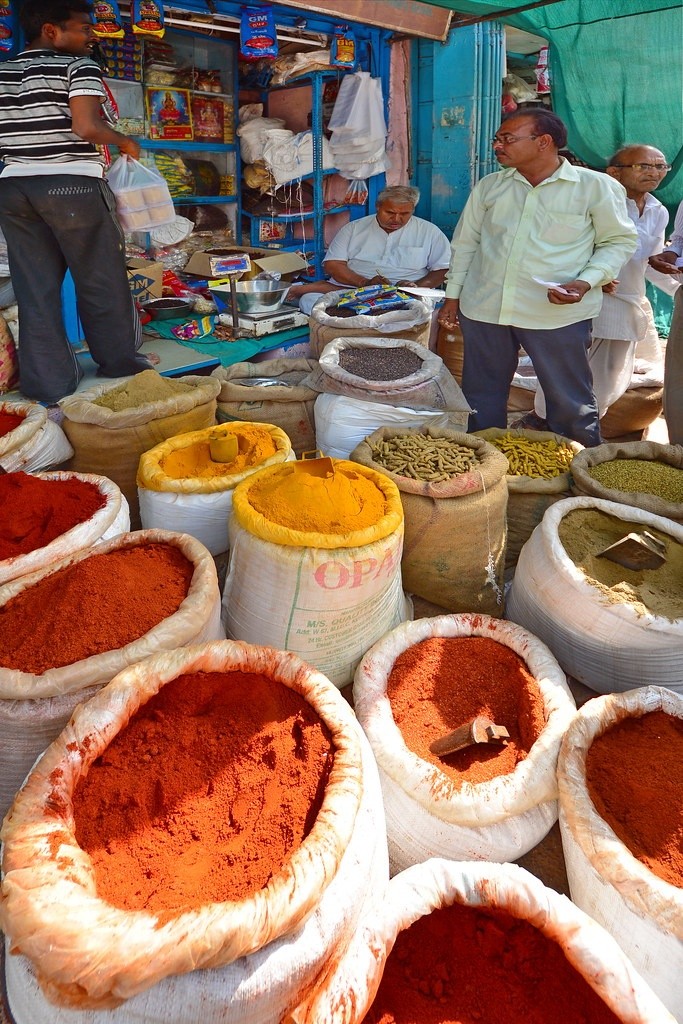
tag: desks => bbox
[0,327,310,407]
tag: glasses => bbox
[614,163,672,173]
[490,135,536,143]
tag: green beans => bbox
[486,432,574,480]
[365,433,480,482]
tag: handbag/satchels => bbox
[107,152,178,233]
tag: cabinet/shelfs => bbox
[101,26,242,246]
[242,71,378,284]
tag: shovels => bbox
[596,531,667,571]
[294,450,336,478]
[429,717,510,757]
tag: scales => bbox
[209,253,310,337]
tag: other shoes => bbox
[511,410,548,431]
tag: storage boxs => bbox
[183,245,311,282]
[126,258,164,304]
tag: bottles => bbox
[211,81,222,93]
[200,82,211,91]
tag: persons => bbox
[648,200,683,448]
[0,0,160,405]
[438,108,638,445]
[517,144,683,433]
[285,186,450,314]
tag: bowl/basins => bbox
[139,297,193,320]
